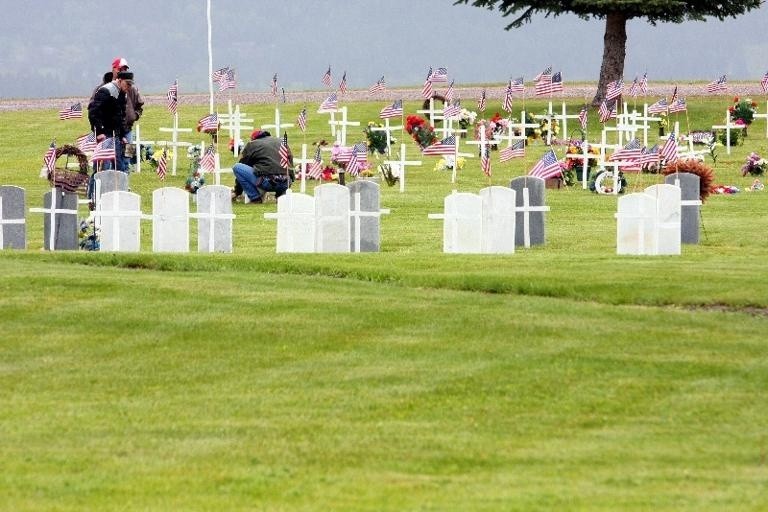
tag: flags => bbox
[499,139,524,163]
[156,62,461,183]
[481,144,491,178]
[478,64,647,128]
[609,130,677,172]
[528,148,561,182]
[647,86,686,113]
[706,73,727,93]
[761,72,767,92]
[40,100,115,179]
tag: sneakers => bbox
[247,200,262,204]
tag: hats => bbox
[117,72,135,84]
[112,57,130,69]
[250,130,271,140]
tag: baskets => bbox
[48,145,90,193]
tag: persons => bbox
[231,129,296,205]
[87,71,136,212]
[89,58,145,192]
[88,72,112,101]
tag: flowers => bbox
[365,108,620,198]
[182,144,207,191]
[724,88,768,176]
